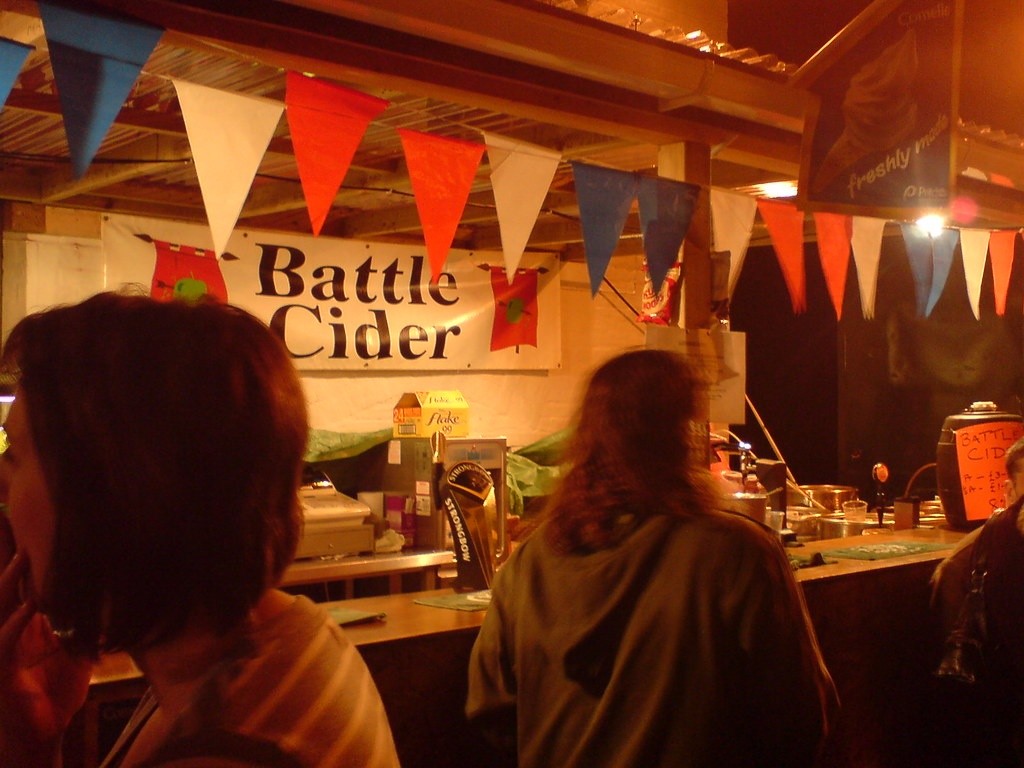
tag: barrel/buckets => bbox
[936,401,1024,531]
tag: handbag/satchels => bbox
[922,521,1007,768]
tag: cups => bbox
[770,511,783,532]
[841,499,868,521]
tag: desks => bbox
[280,538,981,768]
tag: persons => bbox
[462,346,843,768]
[1,282,402,768]
[927,441,1024,768]
